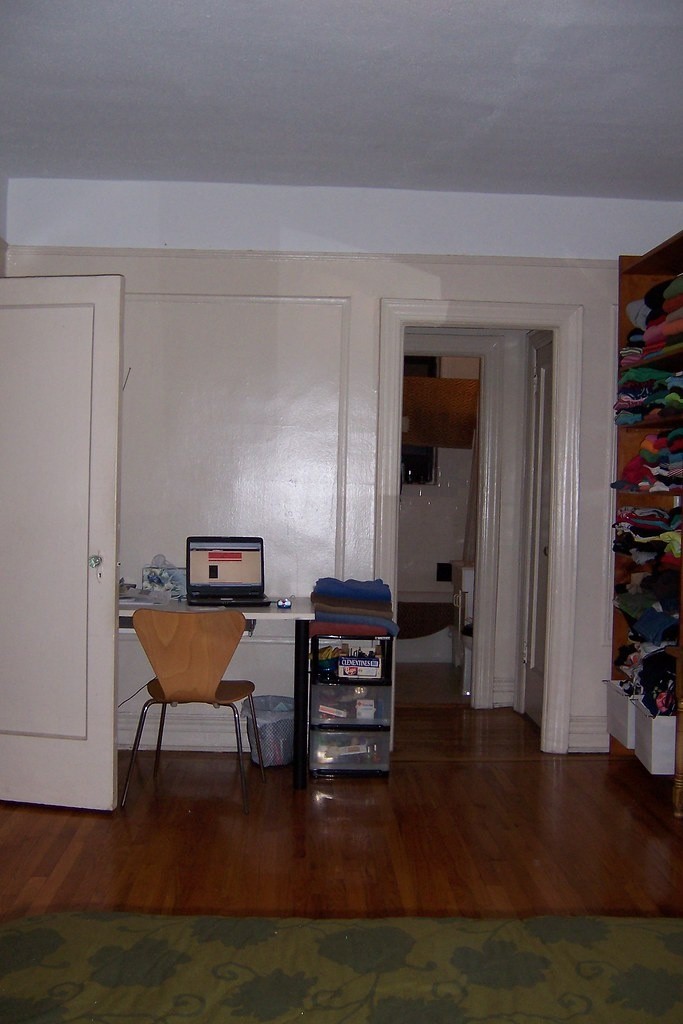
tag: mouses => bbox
[277,599,292,609]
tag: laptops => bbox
[186,535,271,607]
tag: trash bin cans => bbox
[242,695,295,767]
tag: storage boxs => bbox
[634,698,676,775]
[605,680,644,749]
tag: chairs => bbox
[120,609,265,816]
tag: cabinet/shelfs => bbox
[293,618,396,783]
[451,561,476,695]
[610,228,683,817]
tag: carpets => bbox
[0,914,683,1024]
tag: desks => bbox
[118,596,315,782]
